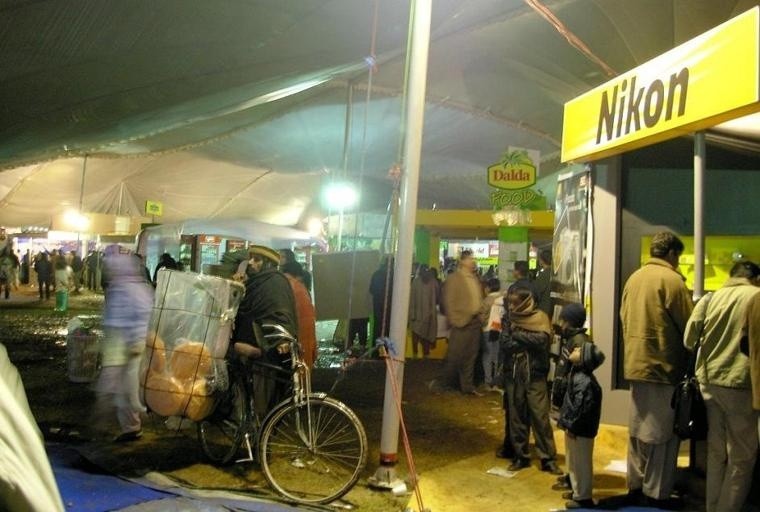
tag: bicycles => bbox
[192,319,370,510]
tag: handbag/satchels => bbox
[670,376,709,441]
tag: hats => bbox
[560,302,587,329]
[249,245,280,263]
[580,342,605,373]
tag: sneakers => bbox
[552,474,595,509]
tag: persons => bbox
[683,260,760,512]
[97,245,315,440]
[348,249,605,508]
[1,243,105,298]
[739,291,760,442]
[620,231,694,509]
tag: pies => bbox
[144,331,218,421]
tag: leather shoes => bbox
[541,461,563,475]
[507,456,531,471]
